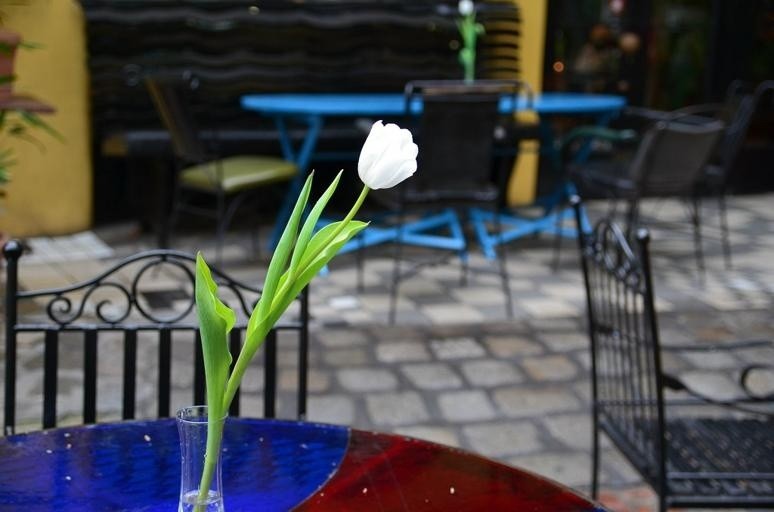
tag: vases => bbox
[175,405,229,512]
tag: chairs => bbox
[2,240,308,435]
[570,195,774,512]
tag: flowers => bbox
[192,118,419,512]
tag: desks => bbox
[0,416,612,511]
